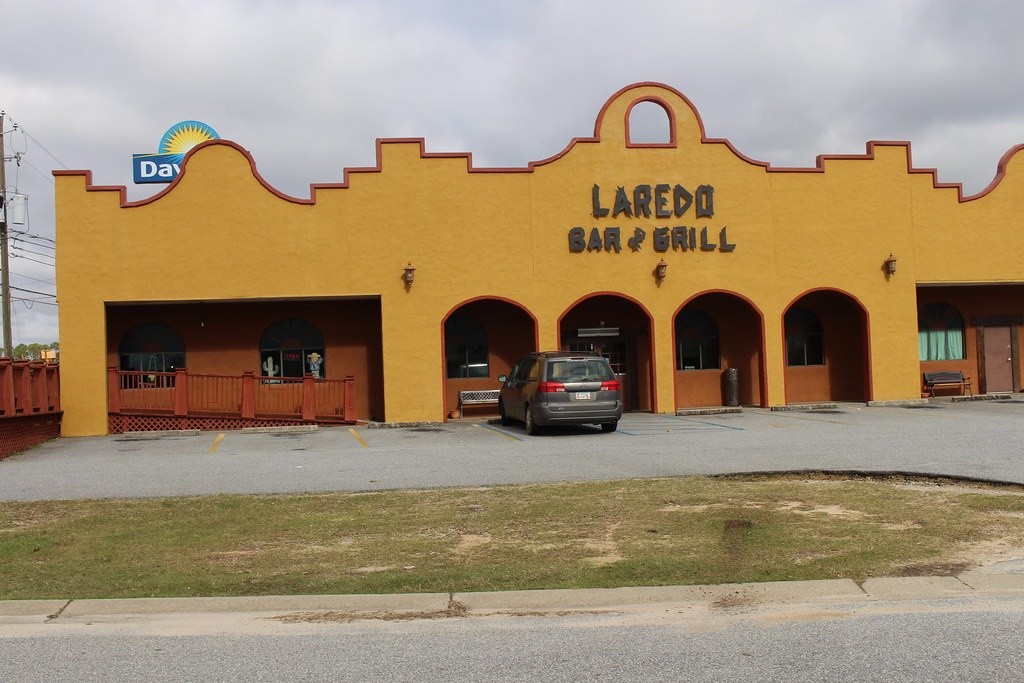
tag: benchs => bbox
[458,390,501,418]
[924,370,973,398]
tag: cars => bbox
[497,350,621,434]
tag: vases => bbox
[922,393,930,397]
[450,410,459,419]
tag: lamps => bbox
[657,258,667,278]
[404,262,416,284]
[888,253,895,274]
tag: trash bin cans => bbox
[725,368,740,407]
